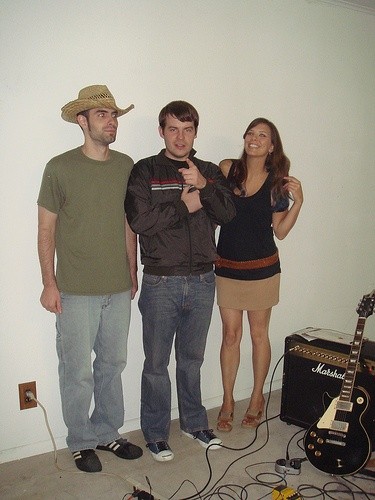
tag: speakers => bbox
[280,333,375,452]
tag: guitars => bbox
[303,287,375,477]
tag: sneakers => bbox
[96,438,143,459]
[73,450,102,472]
[146,441,175,462]
[182,429,224,450]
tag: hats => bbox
[60,85,134,124]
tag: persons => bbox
[37,85,143,472]
[217,118,304,433]
[124,101,237,461]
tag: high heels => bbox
[217,400,236,432]
[241,397,265,428]
